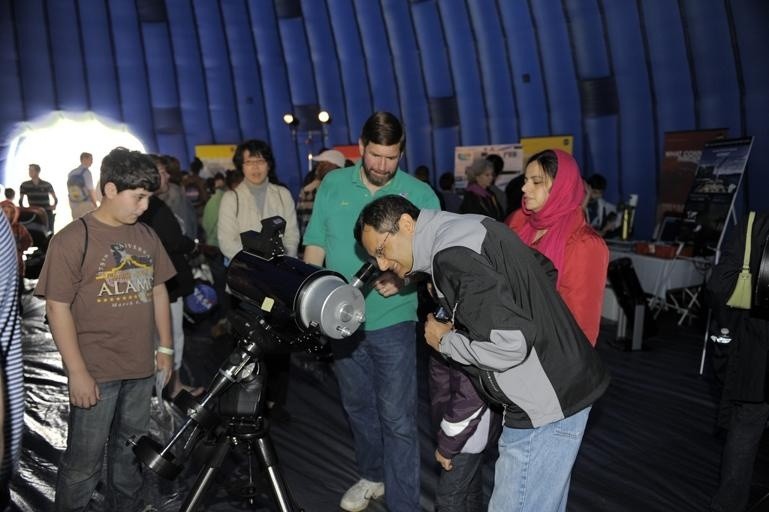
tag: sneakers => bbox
[340,479,384,512]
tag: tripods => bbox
[178,419,301,512]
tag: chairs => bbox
[640,210,718,331]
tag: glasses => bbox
[376,218,399,256]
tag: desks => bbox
[590,233,717,352]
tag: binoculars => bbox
[126,215,378,483]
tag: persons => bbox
[296,111,624,512]
[1,140,301,512]
[706,206,768,512]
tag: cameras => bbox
[434,305,452,324]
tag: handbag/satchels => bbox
[726,269,752,309]
[183,245,226,329]
